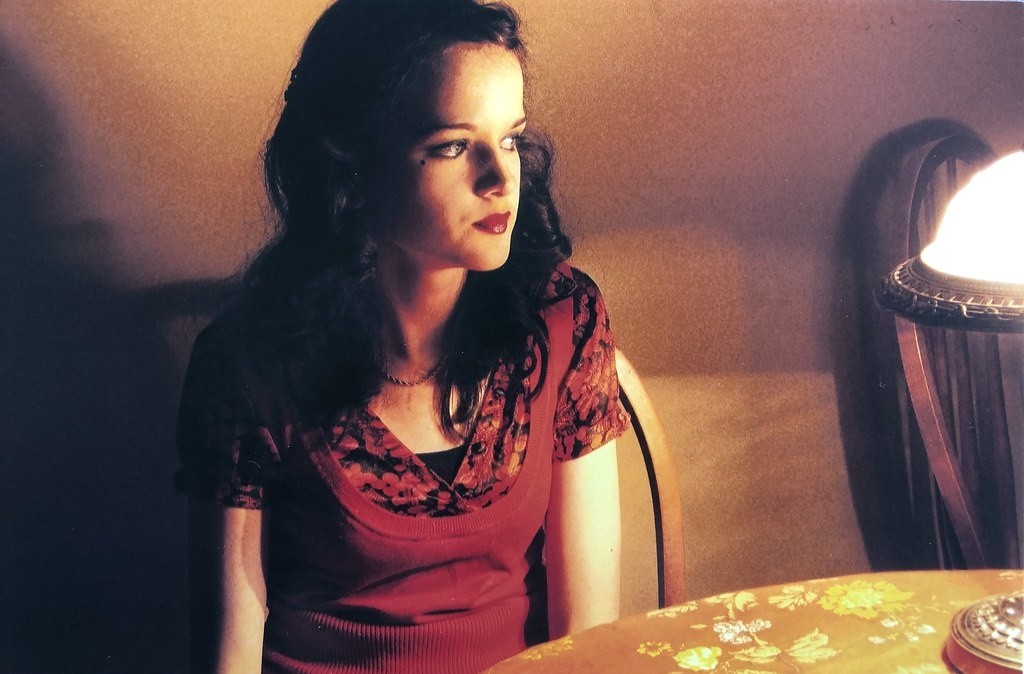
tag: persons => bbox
[187,2,628,671]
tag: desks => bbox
[474,566,1024,672]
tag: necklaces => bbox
[370,346,451,387]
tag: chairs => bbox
[882,134,1021,569]
[528,346,685,652]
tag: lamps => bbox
[877,149,1024,673]
